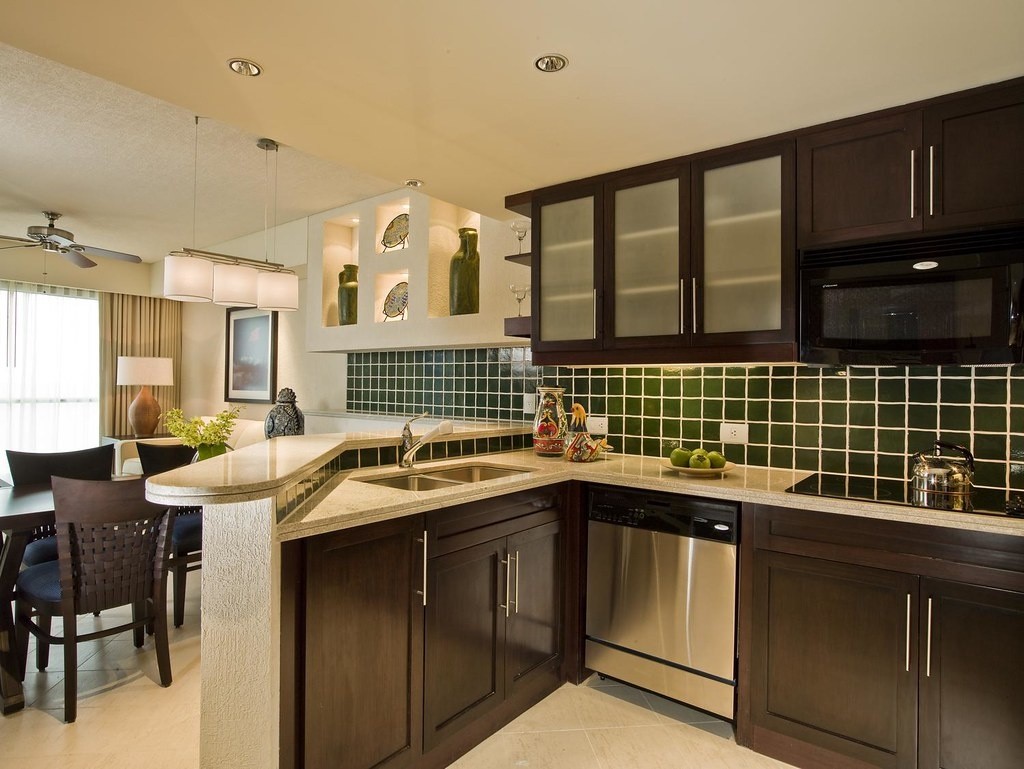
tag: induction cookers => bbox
[783,473,1024,518]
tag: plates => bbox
[384,282,408,318]
[383,213,409,248]
[661,460,736,477]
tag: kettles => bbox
[911,440,976,494]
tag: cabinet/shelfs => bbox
[505,76,1024,365]
[279,479,572,769]
[736,503,1024,769]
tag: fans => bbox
[0,211,142,268]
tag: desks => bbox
[0,475,143,714]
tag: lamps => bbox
[165,115,299,311]
[117,356,174,440]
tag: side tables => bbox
[103,433,177,476]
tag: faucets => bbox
[399,411,454,468]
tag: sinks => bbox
[348,470,469,492]
[417,460,544,485]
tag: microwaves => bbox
[797,226,1024,369]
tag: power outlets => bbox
[524,394,536,414]
[719,423,749,445]
[586,417,609,435]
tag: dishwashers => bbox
[583,486,740,730]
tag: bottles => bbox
[532,387,568,458]
[449,227,479,316]
[338,265,357,325]
[564,403,597,461]
[264,387,304,440]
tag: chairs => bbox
[0,441,198,723]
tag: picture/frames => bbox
[224,307,278,404]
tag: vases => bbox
[449,228,479,315]
[338,265,358,325]
[534,386,569,457]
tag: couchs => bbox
[116,416,265,477]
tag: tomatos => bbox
[670,447,726,470]
